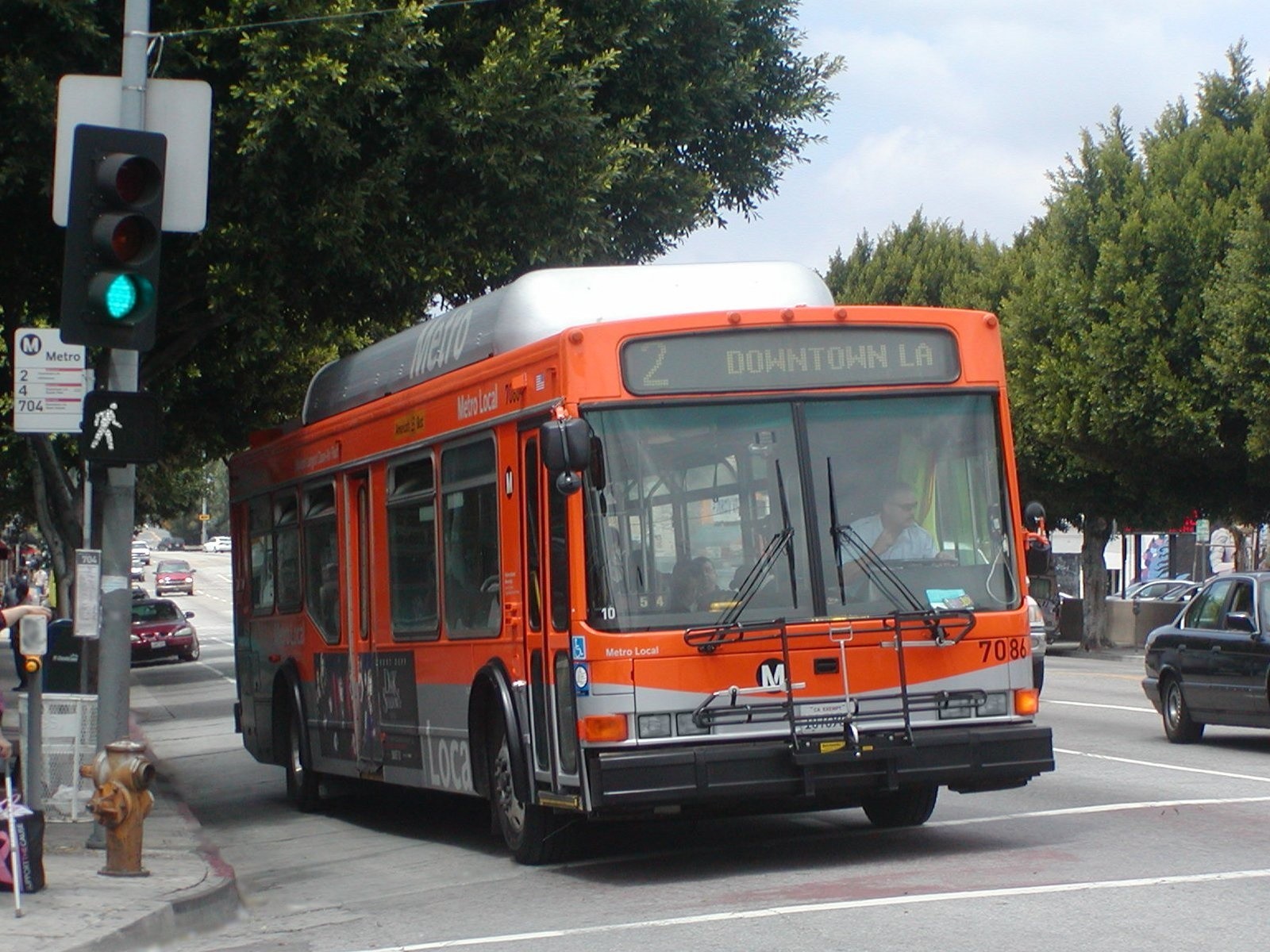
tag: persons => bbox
[606,525,673,612]
[32,554,49,599]
[412,549,499,634]
[11,583,33,694]
[834,482,937,603]
[262,552,313,614]
[667,557,785,613]
[0,605,52,760]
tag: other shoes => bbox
[12,681,27,691]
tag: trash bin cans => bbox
[18,693,97,822]
[42,617,83,694]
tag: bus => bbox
[227,260,1056,867]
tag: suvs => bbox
[943,541,1048,699]
[131,540,152,565]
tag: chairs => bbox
[671,560,696,613]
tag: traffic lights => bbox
[81,390,161,464]
[59,123,167,353]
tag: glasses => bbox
[890,502,917,511]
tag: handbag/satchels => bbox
[0,794,46,894]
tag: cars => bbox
[130,584,148,600]
[130,598,200,662]
[130,556,146,582]
[203,536,232,553]
[153,560,197,596]
[1142,571,1270,744]
[157,537,185,551]
[1114,578,1203,601]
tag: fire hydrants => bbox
[80,734,156,877]
